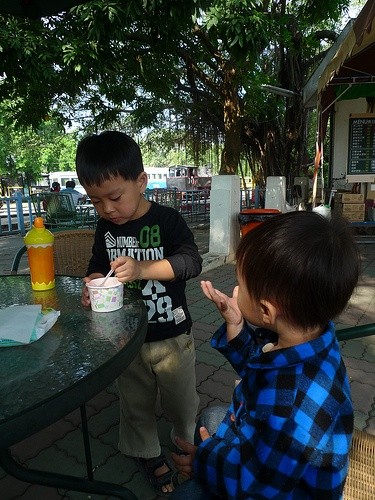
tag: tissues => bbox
[0,303,62,349]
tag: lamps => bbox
[261,83,303,99]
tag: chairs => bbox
[9,229,375,500]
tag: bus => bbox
[49,170,87,196]
[144,167,171,190]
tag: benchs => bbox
[29,191,96,229]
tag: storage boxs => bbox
[332,191,365,222]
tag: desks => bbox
[0,274,148,500]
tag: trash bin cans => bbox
[239,208,280,239]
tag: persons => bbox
[168,210,361,500]
[76,131,203,495]
[43,182,61,211]
[58,181,86,211]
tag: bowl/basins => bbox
[85,277,124,313]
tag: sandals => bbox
[138,455,177,497]
[167,435,190,455]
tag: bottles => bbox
[23,217,55,291]
[312,204,331,220]
